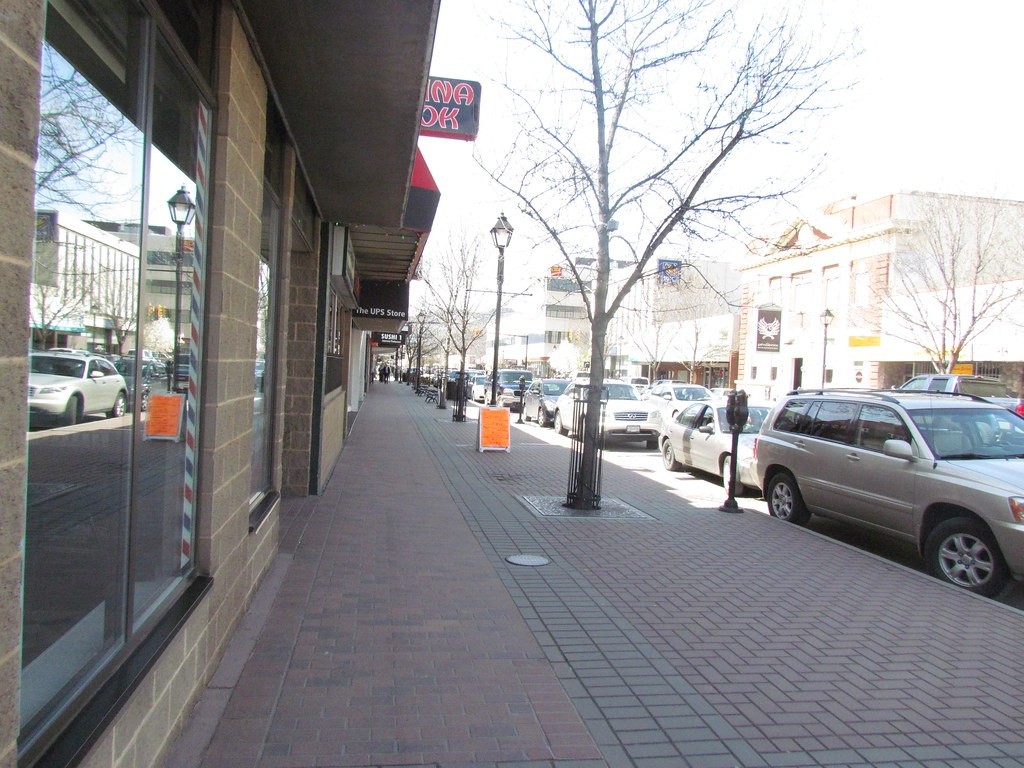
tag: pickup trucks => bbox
[899,373,1024,421]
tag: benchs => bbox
[417,385,438,403]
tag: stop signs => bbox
[855,371,863,382]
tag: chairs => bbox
[544,385,550,396]
[863,415,889,451]
[931,415,973,455]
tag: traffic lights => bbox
[719,370,724,376]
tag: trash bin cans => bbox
[447,381,456,400]
[403,373,408,382]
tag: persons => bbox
[379,364,390,384]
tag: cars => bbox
[522,378,571,427]
[409,362,491,403]
[657,399,779,498]
[618,376,737,452]
[26,344,190,431]
[254,349,266,392]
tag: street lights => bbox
[489,211,514,407]
[166,185,197,402]
[819,308,835,387]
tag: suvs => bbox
[553,377,659,451]
[568,370,591,382]
[754,388,1024,598]
[483,368,535,412]
